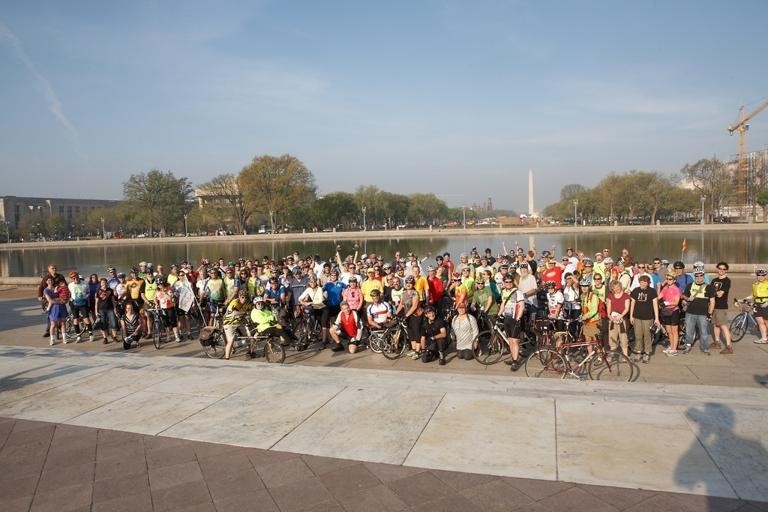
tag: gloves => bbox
[760,302,768,308]
[706,313,713,322]
[336,329,341,335]
[735,300,742,306]
[515,320,520,328]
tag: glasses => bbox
[718,268,727,270]
[757,274,766,277]
[52,268,56,269]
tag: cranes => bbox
[727,95,768,223]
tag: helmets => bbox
[611,312,623,324]
[650,324,660,334]
[756,269,767,275]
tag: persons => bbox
[709,262,734,354]
[38,241,711,371]
[742,269,767,343]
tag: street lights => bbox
[699,194,707,225]
[361,206,367,231]
[100,216,106,240]
[572,199,579,227]
[268,210,275,236]
[461,207,466,229]
[182,213,190,238]
[4,221,11,243]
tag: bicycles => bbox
[524,323,634,382]
[39,295,330,364]
[362,302,712,365]
[727,297,768,342]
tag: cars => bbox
[323,227,333,232]
[629,219,645,225]
[137,232,157,238]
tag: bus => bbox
[258,224,272,233]
